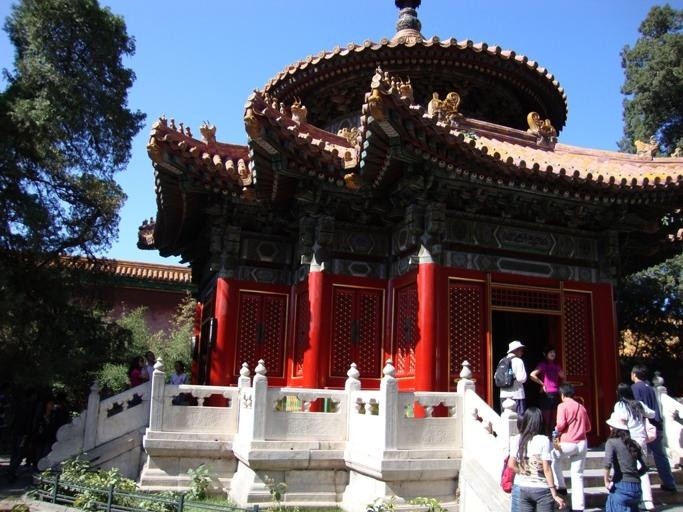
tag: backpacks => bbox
[494,355,521,388]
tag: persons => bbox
[529,347,568,434]
[506,413,524,512]
[603,412,650,512]
[6,387,69,485]
[516,405,567,512]
[169,361,190,404]
[500,340,528,416]
[141,350,156,381]
[128,355,149,387]
[630,364,678,492]
[550,383,592,512]
[613,383,657,511]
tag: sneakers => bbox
[660,484,678,492]
[555,488,567,496]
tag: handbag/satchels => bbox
[642,417,658,443]
[500,456,518,493]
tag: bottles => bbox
[552,431,559,450]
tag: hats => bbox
[605,411,630,432]
[507,339,525,354]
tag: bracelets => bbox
[547,485,556,488]
